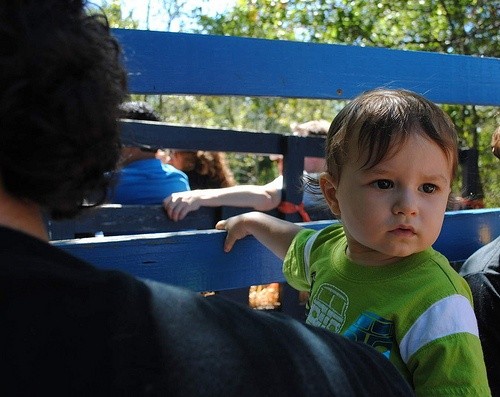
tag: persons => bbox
[216,88,490,397]
[107,101,191,205]
[1,1,413,397]
[166,146,250,305]
[269,154,284,174]
[457,125,500,392]
[163,120,336,304]
